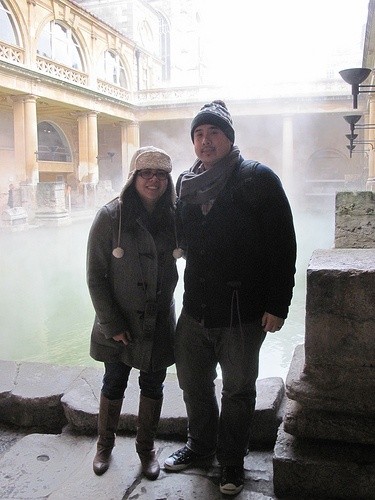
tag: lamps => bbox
[339,68,375,158]
[35,145,57,163]
[96,152,115,165]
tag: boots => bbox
[135,395,164,480]
[92,391,125,475]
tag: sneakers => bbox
[219,465,245,495]
[163,446,217,471]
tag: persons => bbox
[86,146,179,477]
[163,100,297,494]
[7,184,14,209]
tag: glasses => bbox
[136,171,169,181]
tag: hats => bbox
[118,146,177,209]
[190,99,235,144]
